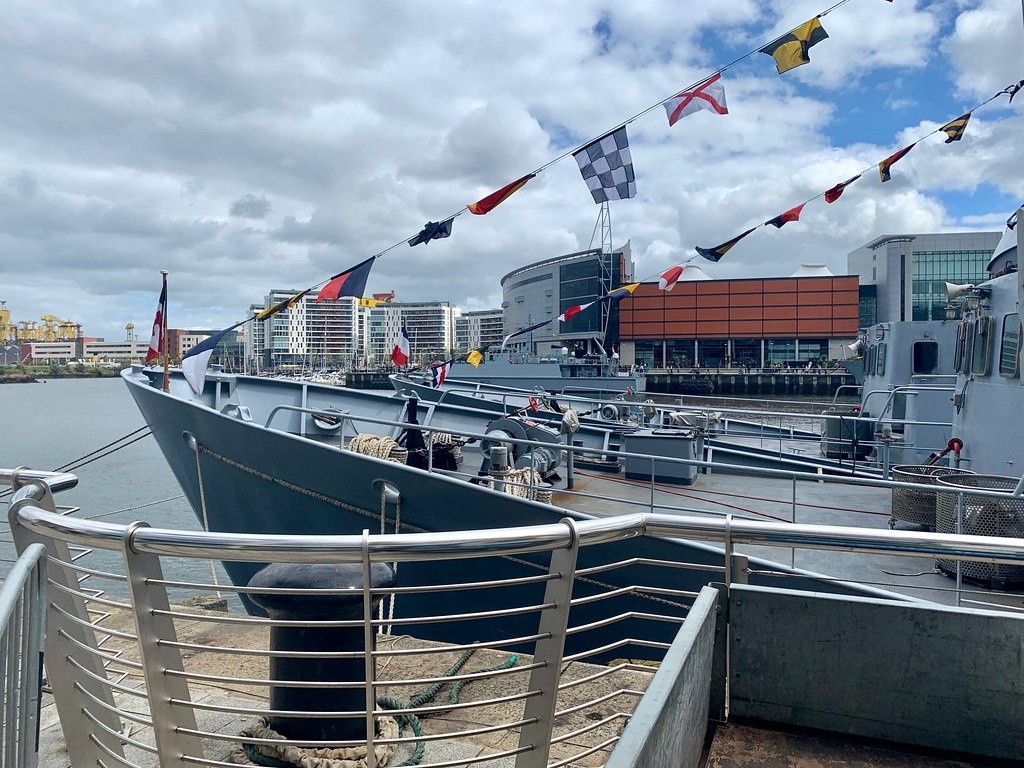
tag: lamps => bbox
[965,291,987,310]
[876,326,884,341]
[944,304,963,319]
[858,330,864,341]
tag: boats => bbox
[112,194,1023,669]
[386,316,962,442]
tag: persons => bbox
[617,358,850,375]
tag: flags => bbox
[500,321,552,350]
[938,108,976,144]
[145,279,166,364]
[824,174,863,203]
[317,255,376,306]
[695,227,756,263]
[1003,80,1024,104]
[407,217,454,246]
[558,302,595,322]
[758,14,829,75]
[662,71,730,128]
[181,324,234,397]
[572,127,641,204]
[431,359,455,388]
[456,341,495,369]
[601,281,645,303]
[659,260,692,291]
[764,201,810,229]
[254,290,309,322]
[390,322,410,370]
[880,144,916,183]
[466,172,536,215]
